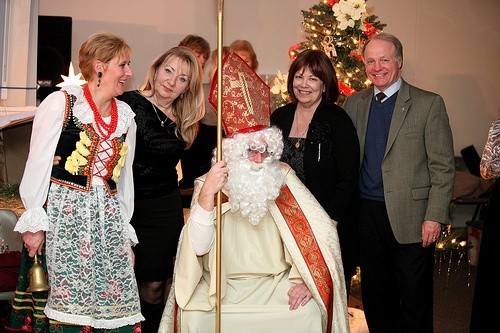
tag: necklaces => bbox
[295,110,308,148]
[152,103,172,127]
[84,84,118,140]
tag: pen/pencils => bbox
[317,142,321,162]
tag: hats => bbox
[207,48,271,139]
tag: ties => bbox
[376,91,387,103]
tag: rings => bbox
[433,236,437,239]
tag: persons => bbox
[115,47,205,333]
[469,118,500,332]
[12,32,145,333]
[157,51,349,333]
[342,33,454,333]
[178,34,258,184]
[269,49,360,307]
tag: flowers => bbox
[332,0,366,30]
